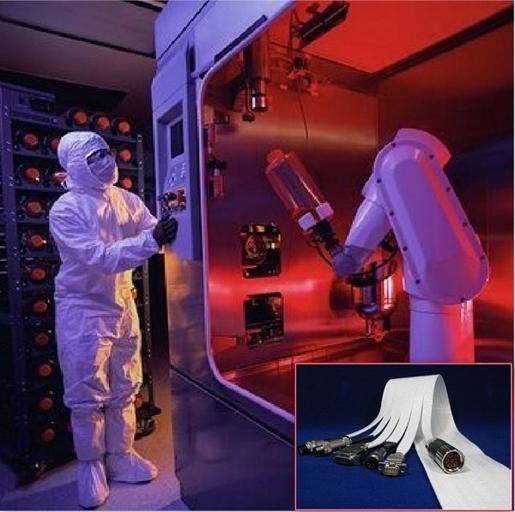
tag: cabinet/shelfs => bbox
[0,81,161,487]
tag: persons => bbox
[48,131,179,511]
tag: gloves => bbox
[153,189,185,247]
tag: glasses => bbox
[87,149,117,164]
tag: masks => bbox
[88,154,119,184]
